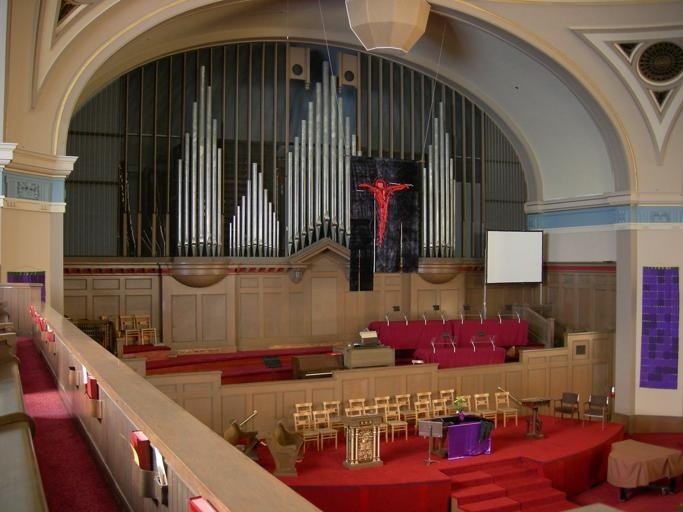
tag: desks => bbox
[368,320,529,368]
[522,398,550,439]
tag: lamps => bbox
[345,0,431,57]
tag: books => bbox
[188,495,219,511]
[129,428,151,471]
[28,303,54,343]
[85,374,97,399]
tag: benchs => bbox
[0,312,49,512]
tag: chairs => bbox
[293,389,518,454]
[554,392,580,425]
[101,315,157,346]
[583,394,609,432]
